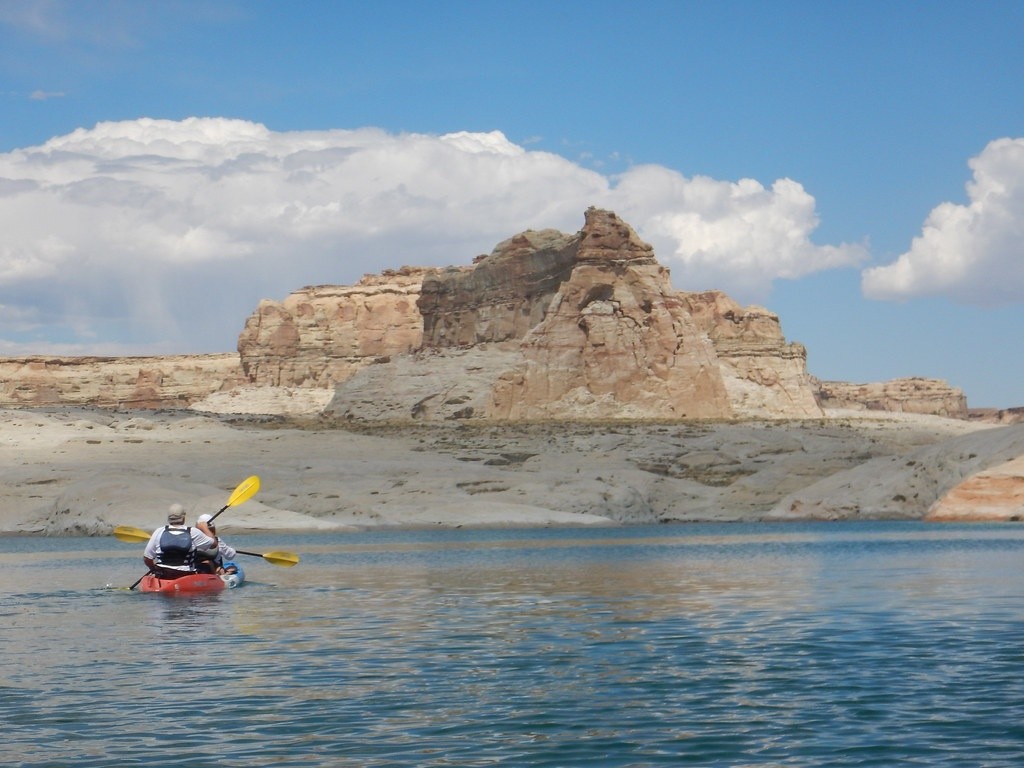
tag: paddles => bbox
[114,524,299,568]
[127,474,261,591]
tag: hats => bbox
[168,504,186,523]
[197,514,215,528]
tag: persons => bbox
[144,504,237,578]
[195,514,236,566]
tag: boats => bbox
[141,561,245,593]
